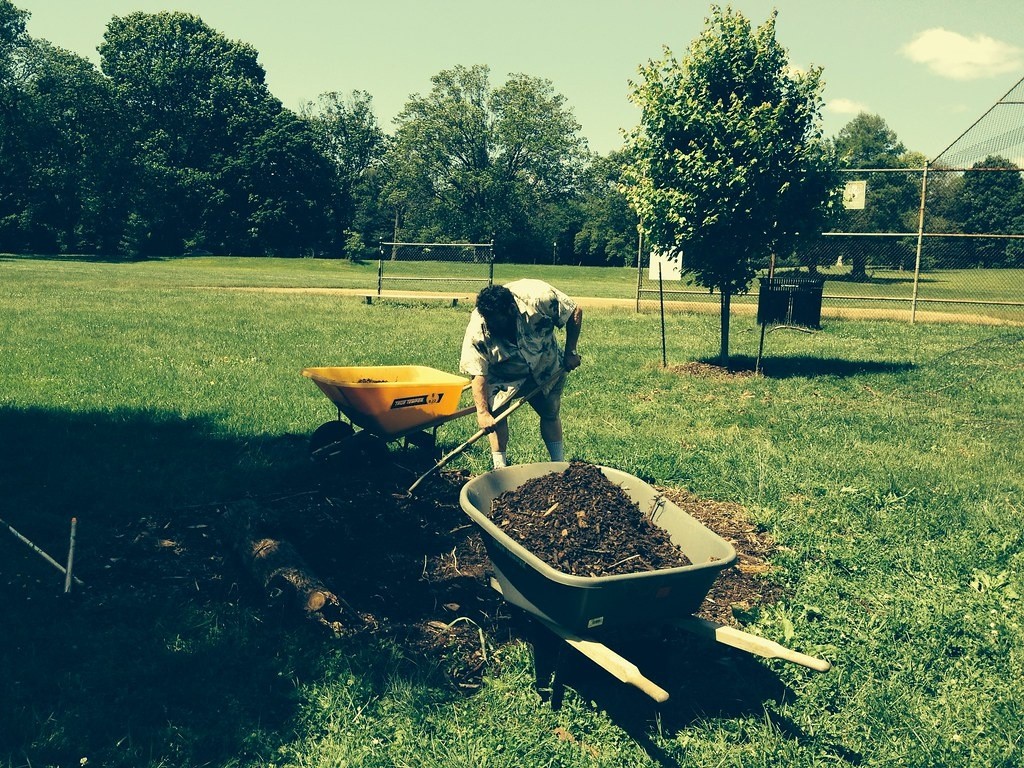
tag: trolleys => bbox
[302,365,477,470]
[458,461,829,702]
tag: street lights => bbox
[553,241,556,265]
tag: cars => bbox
[461,247,496,263]
[183,249,213,257]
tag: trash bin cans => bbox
[757,277,827,329]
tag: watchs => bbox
[564,350,578,357]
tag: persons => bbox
[459,278,583,470]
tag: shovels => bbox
[385,355,581,497]
